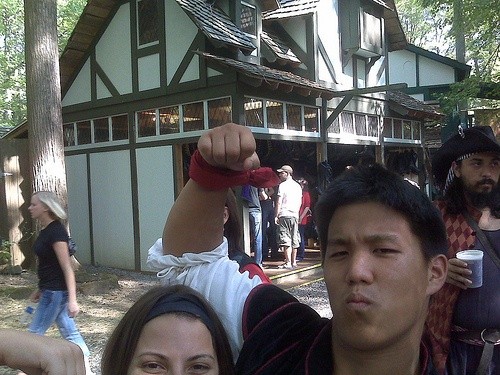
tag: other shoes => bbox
[292,264,297,268]
[278,263,291,269]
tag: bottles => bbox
[18,299,37,326]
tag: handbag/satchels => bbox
[67,236,76,258]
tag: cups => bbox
[456,250,484,289]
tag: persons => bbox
[273,164,303,270]
[146,123,451,375]
[222,187,273,286]
[26,191,91,374]
[422,124,500,375]
[0,282,233,374]
[244,176,334,270]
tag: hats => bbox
[431,125,500,201]
[276,165,293,174]
